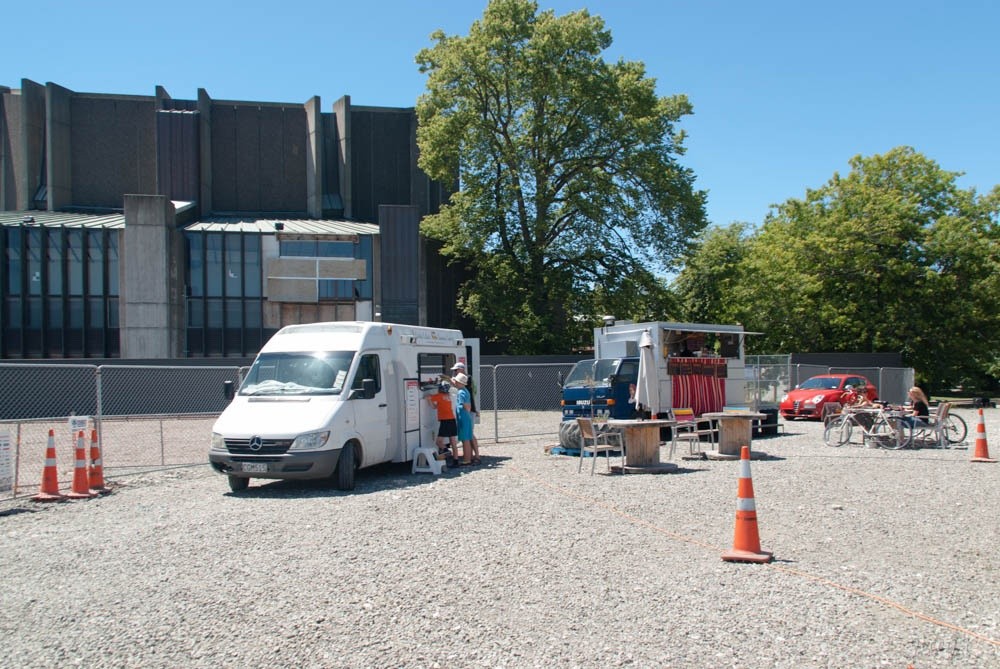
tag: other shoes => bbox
[472,456,482,466]
[460,461,471,466]
[447,460,459,468]
[439,447,450,455]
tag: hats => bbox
[438,380,451,392]
[451,362,465,370]
[453,373,468,386]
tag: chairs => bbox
[912,403,952,449]
[576,417,625,476]
[824,402,843,442]
[666,407,714,460]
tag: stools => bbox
[412,447,445,475]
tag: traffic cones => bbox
[60,430,99,500]
[30,428,64,501]
[85,430,114,493]
[721,446,775,564]
[969,408,999,463]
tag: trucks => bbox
[553,321,781,445]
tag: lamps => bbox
[275,223,285,231]
[22,215,35,223]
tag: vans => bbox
[207,320,480,491]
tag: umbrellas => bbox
[634,330,662,420]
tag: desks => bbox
[607,419,677,474]
[843,409,913,449]
[702,411,767,460]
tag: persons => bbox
[424,362,481,468]
[840,387,884,440]
[889,387,929,445]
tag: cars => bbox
[780,373,880,421]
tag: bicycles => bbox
[940,413,968,444]
[821,399,911,450]
